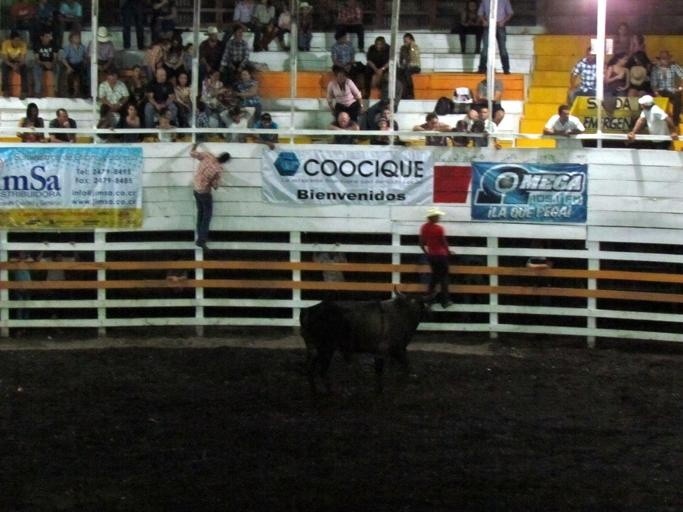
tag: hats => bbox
[97,27,112,43]
[204,26,220,35]
[656,51,672,59]
[630,66,647,85]
[423,208,446,217]
[300,2,312,13]
[638,95,654,107]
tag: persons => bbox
[459,240,487,304]
[525,246,553,325]
[188,141,231,252]
[543,21,683,149]
[313,240,351,302]
[38,239,78,338]
[166,257,188,294]
[420,207,460,308]
[452,0,514,74]
[8,248,35,336]
[1,0,421,147]
[412,70,505,150]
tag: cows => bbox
[300,285,437,398]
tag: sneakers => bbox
[442,301,454,307]
[196,240,210,251]
